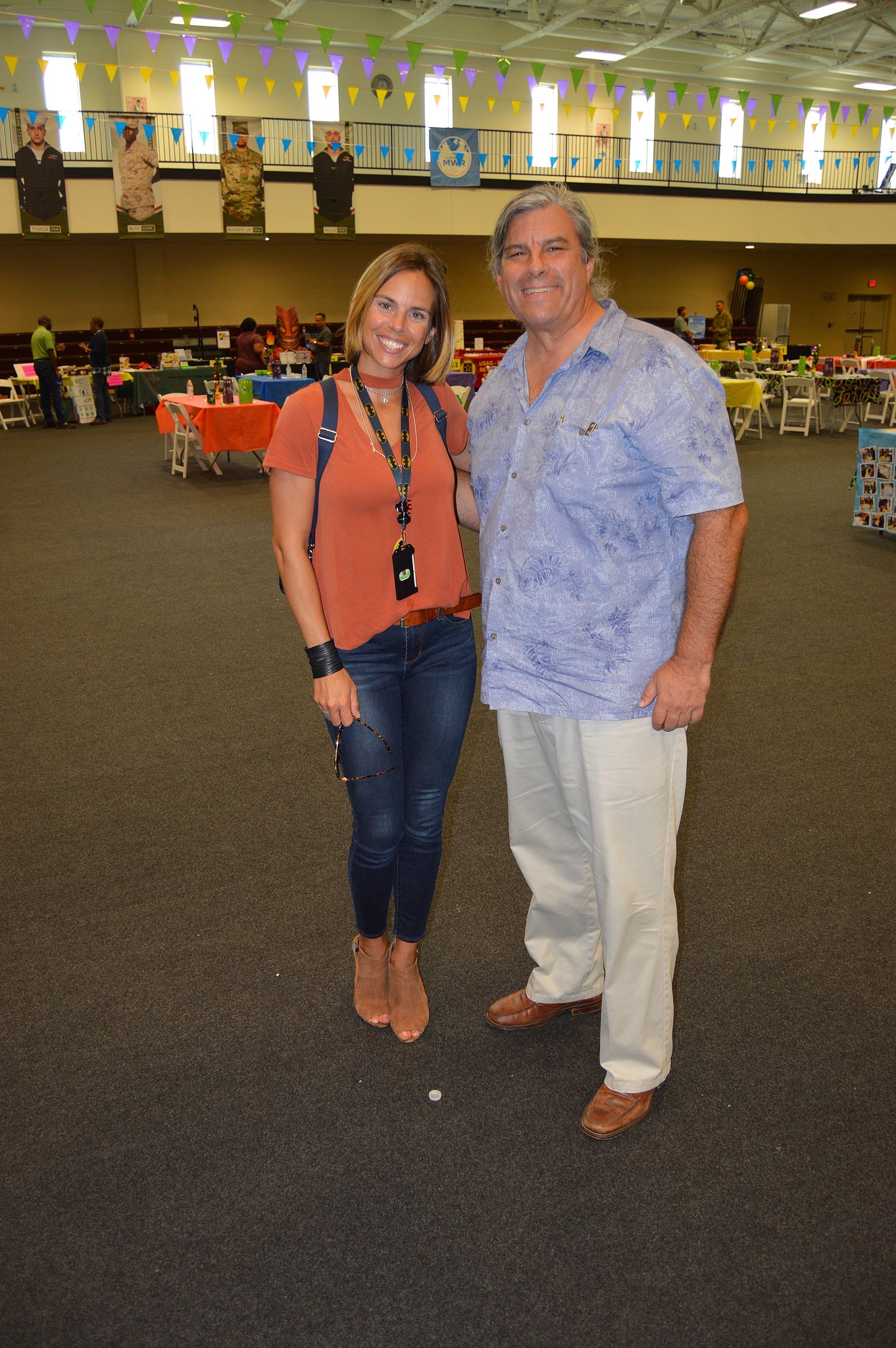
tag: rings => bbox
[321,708,332,720]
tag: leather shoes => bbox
[579,1081,665,1140]
[485,987,603,1030]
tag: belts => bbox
[392,592,482,628]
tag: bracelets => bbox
[305,637,344,680]
[85,348,88,352]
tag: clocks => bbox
[372,74,393,100]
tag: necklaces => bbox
[361,374,405,404]
[348,362,418,468]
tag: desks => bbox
[447,372,477,402]
[807,355,896,373]
[858,368,896,393]
[272,362,348,381]
[738,344,816,363]
[718,377,763,441]
[756,370,882,433]
[119,365,227,416]
[848,428,896,536]
[155,393,281,480]
[187,358,236,372]
[17,370,134,423]
[698,349,772,362]
[236,375,316,406]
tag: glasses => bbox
[334,714,399,782]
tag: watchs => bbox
[313,340,317,345]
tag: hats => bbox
[23,114,48,125]
[321,123,343,134]
[232,122,249,135]
[122,117,140,129]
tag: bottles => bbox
[787,362,792,372]
[286,364,291,379]
[302,365,307,380]
[187,380,194,399]
[303,327,306,335]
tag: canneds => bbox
[160,364,164,371]
[207,391,215,404]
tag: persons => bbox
[15,115,69,222]
[708,300,733,350]
[313,122,354,222]
[673,306,695,348]
[234,317,268,376]
[31,315,77,429]
[115,116,159,221]
[220,122,265,220]
[263,244,476,1043]
[454,181,748,1141]
[305,313,332,381]
[77,316,113,425]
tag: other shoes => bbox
[43,420,56,428]
[56,422,76,429]
[107,416,112,422]
[90,419,107,425]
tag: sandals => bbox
[388,937,429,1043]
[352,933,391,1027]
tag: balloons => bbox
[737,269,756,290]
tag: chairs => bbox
[729,335,896,441]
[0,363,239,478]
